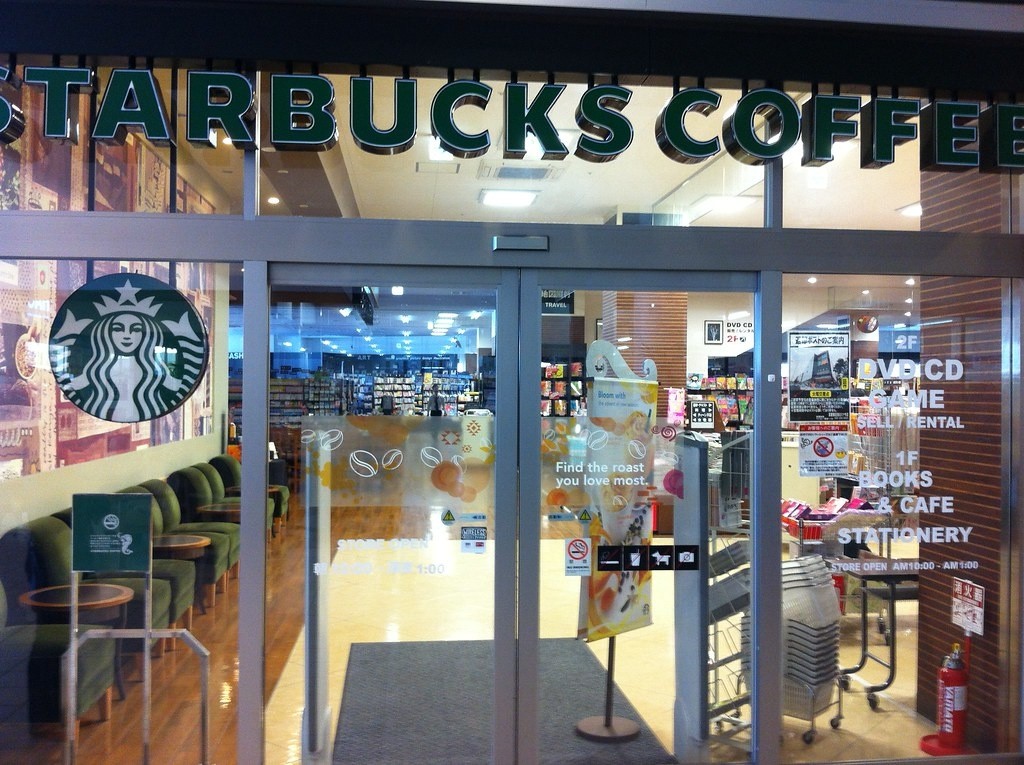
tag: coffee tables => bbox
[18,584,135,701]
[152,534,211,614]
[197,503,241,523]
[226,484,279,538]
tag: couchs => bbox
[0,579,116,765]
[0,516,172,682]
[208,455,290,532]
[167,467,241,577]
[190,463,274,543]
[50,506,196,652]
[137,479,241,591]
[112,485,230,608]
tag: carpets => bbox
[331,637,682,765]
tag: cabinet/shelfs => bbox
[682,371,922,540]
[228,343,594,430]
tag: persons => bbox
[426,383,447,441]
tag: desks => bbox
[823,558,919,710]
